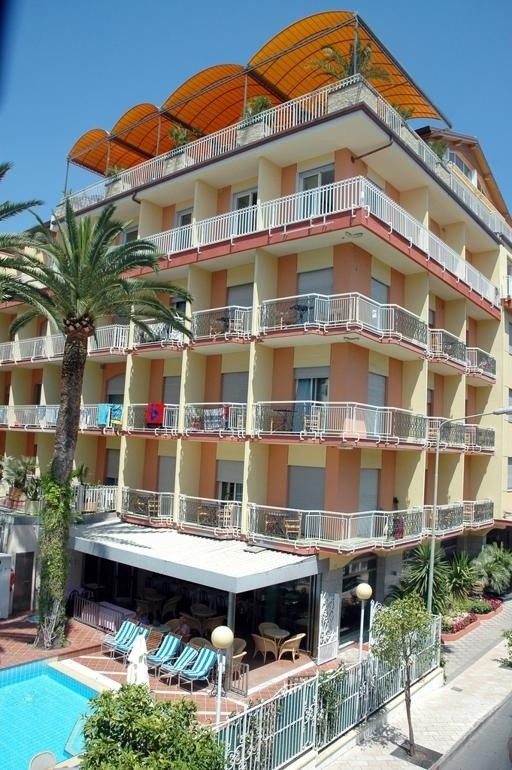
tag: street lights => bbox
[356,583,372,661]
[427,406,512,613]
[211,626,234,727]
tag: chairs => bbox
[148,495,302,541]
[66,576,308,694]
[303,411,320,432]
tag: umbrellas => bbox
[127,632,151,693]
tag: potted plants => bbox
[0,452,101,516]
[104,164,131,198]
[389,104,447,183]
[236,96,274,146]
[305,41,391,115]
[162,122,196,179]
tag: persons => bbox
[128,606,150,626]
[173,616,191,638]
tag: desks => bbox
[274,409,298,431]
[291,303,314,324]
